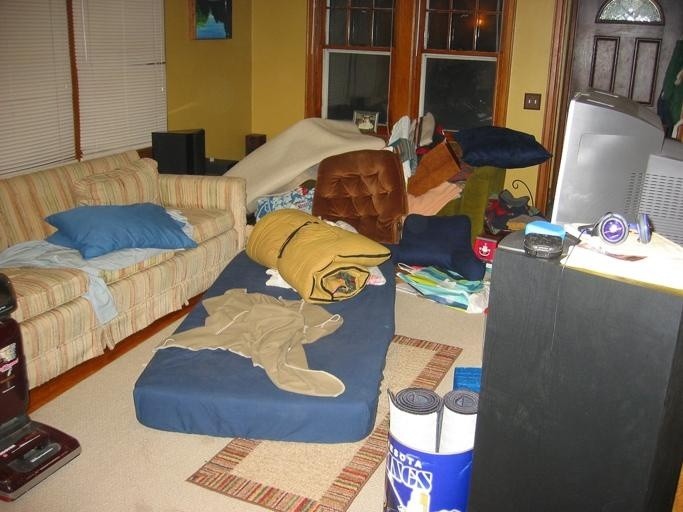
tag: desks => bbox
[205,159,239,177]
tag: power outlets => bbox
[523,93,541,110]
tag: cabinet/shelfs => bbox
[466,228,683,511]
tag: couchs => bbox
[436,140,507,250]
[0,150,247,390]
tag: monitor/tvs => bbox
[548,85,683,248]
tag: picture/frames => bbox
[352,110,379,134]
[188,0,234,40]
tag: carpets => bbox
[0,288,488,512]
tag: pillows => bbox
[46,202,198,259]
[453,124,553,169]
[45,219,186,251]
[72,158,165,208]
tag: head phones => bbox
[578,211,655,245]
